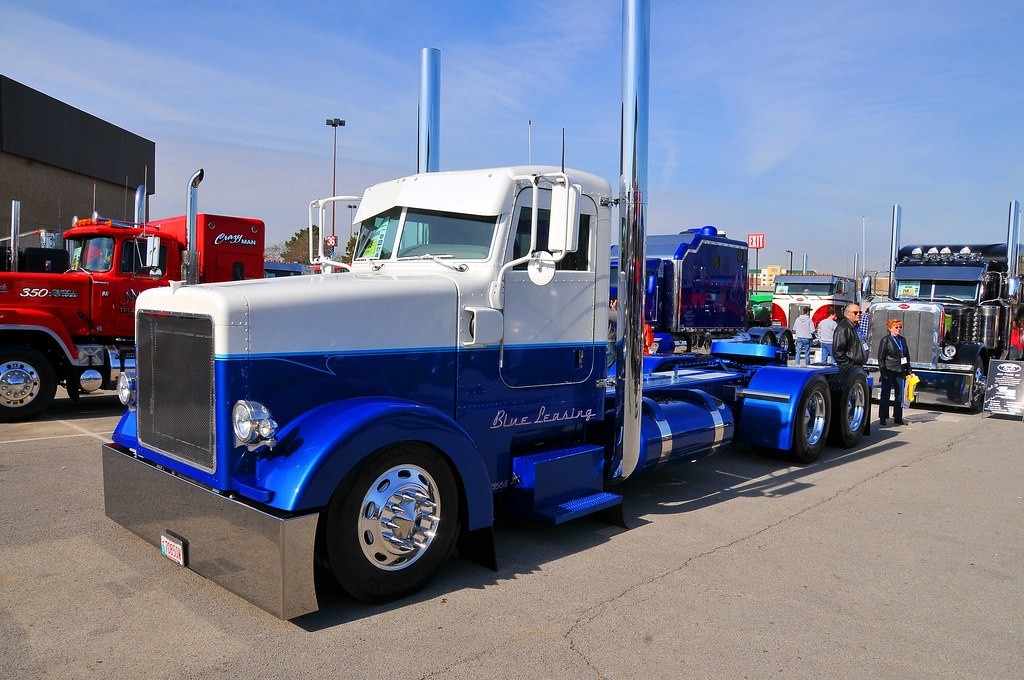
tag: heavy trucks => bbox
[859,199,1024,413]
[0,168,317,422]
[103,1,876,618]
[770,250,891,357]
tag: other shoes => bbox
[880,419,887,425]
[894,417,908,425]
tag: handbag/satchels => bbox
[905,375,920,402]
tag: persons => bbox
[1010,320,1020,346]
[608,298,654,355]
[755,307,770,327]
[878,318,915,425]
[793,304,869,365]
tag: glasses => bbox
[892,326,902,329]
[849,311,862,315]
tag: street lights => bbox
[348,204,358,239]
[324,117,347,257]
[785,249,794,275]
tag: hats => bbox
[803,306,813,311]
